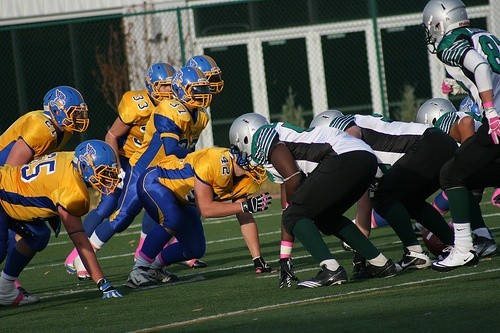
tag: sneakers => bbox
[350,255,397,282]
[394,247,431,273]
[433,245,479,272]
[474,236,499,258]
[296,264,349,288]
[125,265,178,289]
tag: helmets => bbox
[145,62,175,97]
[309,109,344,127]
[72,140,119,184]
[229,112,270,160]
[171,67,210,102]
[460,96,479,115]
[43,86,86,127]
[415,97,457,125]
[422,0,470,43]
[186,55,222,77]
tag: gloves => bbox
[240,192,272,213]
[97,278,123,300]
[484,107,500,144]
[279,257,299,289]
[442,78,465,96]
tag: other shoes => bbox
[255,263,278,275]
[178,259,207,268]
[63,257,92,280]
[0,277,38,306]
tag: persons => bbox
[422,0,500,272]
[0,56,500,307]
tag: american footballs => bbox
[422,232,447,257]
